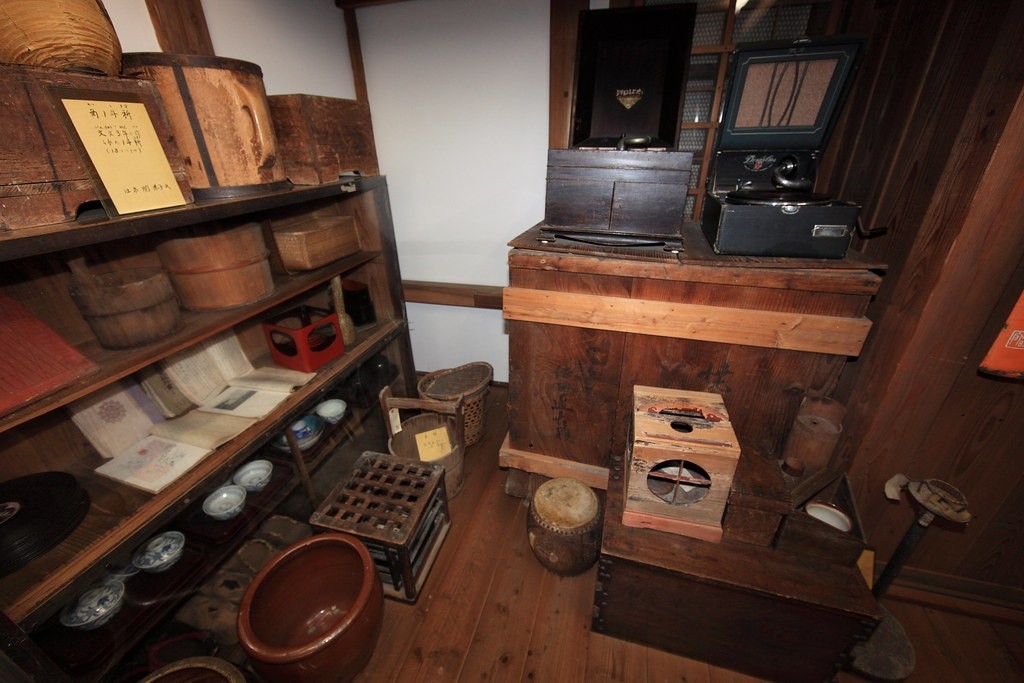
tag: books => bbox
[69,330,316,493]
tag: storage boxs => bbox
[307,450,452,605]
[274,215,362,270]
[586,385,882,683]
[260,304,343,373]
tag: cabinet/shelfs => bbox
[0,174,418,683]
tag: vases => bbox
[136,655,246,683]
[236,533,384,683]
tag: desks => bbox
[498,215,884,508]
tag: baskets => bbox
[418,368,493,447]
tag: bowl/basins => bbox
[807,500,854,534]
[233,460,273,491]
[271,413,324,451]
[316,399,347,423]
[237,533,384,683]
[132,531,185,572]
[138,655,259,683]
[203,485,246,519]
[60,579,126,629]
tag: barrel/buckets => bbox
[155,223,275,311]
[120,52,290,198]
[68,267,184,350]
[379,386,465,500]
